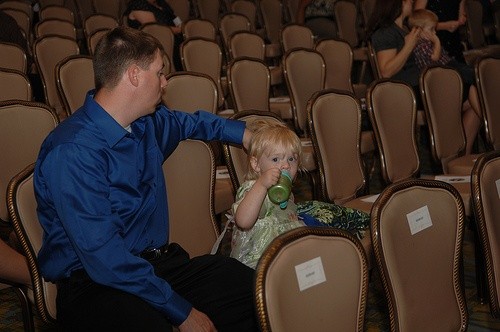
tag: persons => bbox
[365,0,492,153]
[228,119,307,270]
[0,239,33,289]
[32,26,261,332]
[127,0,185,73]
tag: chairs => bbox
[0,0,500,332]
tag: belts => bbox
[138,244,168,261]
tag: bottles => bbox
[268,171,292,203]
